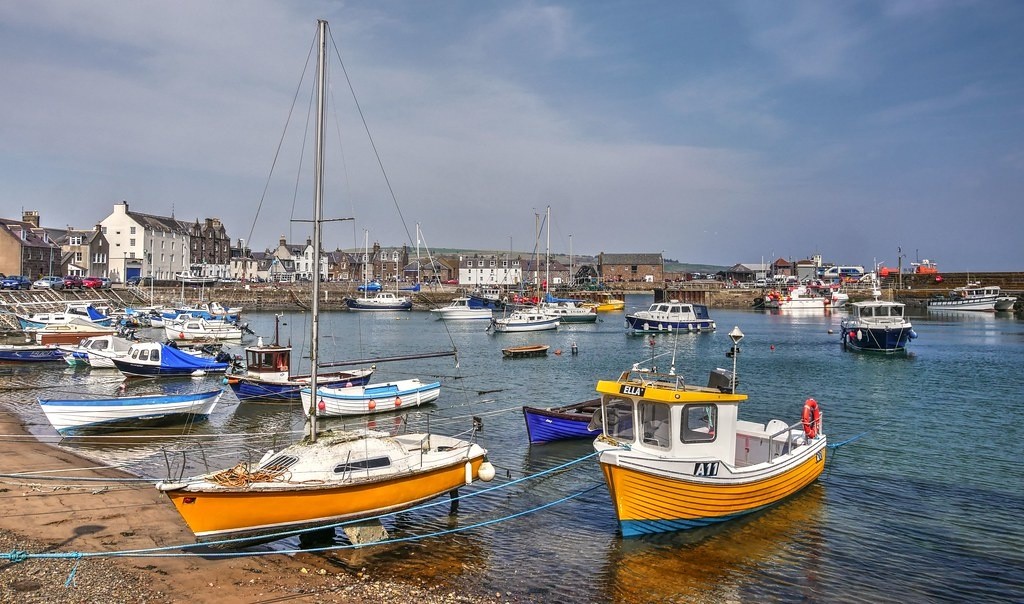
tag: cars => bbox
[1,275,32,290]
[33,276,66,290]
[82,276,102,289]
[98,277,112,289]
[125,276,140,286]
[444,278,458,285]
[357,282,383,293]
[62,275,84,289]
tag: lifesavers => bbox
[800,398,819,440]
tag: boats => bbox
[0,342,67,364]
[521,398,633,447]
[35,384,224,436]
[112,338,242,379]
[580,298,624,312]
[430,295,495,321]
[501,345,550,357]
[298,376,438,416]
[762,279,847,309]
[839,262,918,356]
[60,334,135,368]
[220,315,377,404]
[589,325,829,535]
[960,286,1019,312]
[624,300,715,333]
[15,299,258,349]
[928,294,997,312]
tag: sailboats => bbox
[526,207,597,324]
[343,230,414,312]
[152,16,495,556]
[487,207,560,333]
